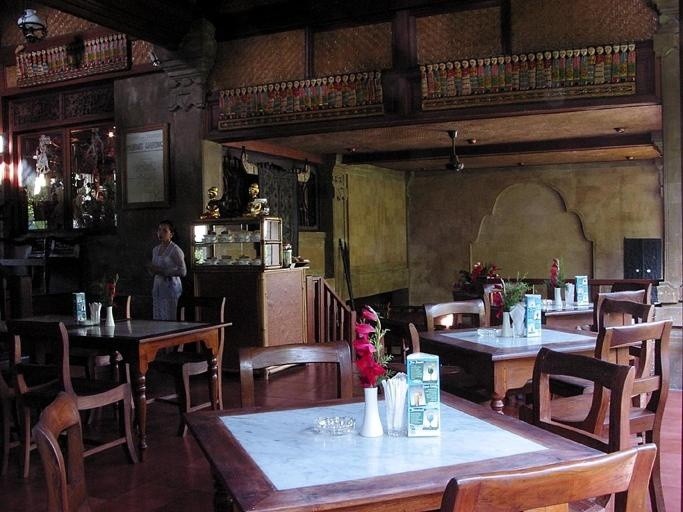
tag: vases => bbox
[105,306,115,327]
[553,287,563,311]
[501,312,511,337]
[359,388,383,438]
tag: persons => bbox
[145,220,186,356]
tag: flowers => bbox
[449,261,500,295]
[95,273,119,306]
[354,304,396,388]
[542,256,568,292]
[492,272,530,318]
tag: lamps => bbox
[17,6,47,43]
[447,130,464,172]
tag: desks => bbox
[194,267,309,379]
[418,324,599,415]
[514,300,594,330]
[66,316,232,461]
[184,386,615,512]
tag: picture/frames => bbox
[121,122,170,209]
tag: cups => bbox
[387,399,408,436]
[512,321,524,336]
[90,310,100,325]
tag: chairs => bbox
[2,330,60,478]
[423,299,486,331]
[522,320,673,512]
[594,283,652,332]
[33,390,136,512]
[439,444,657,512]
[531,347,635,512]
[44,295,136,424]
[145,295,226,439]
[381,318,463,392]
[240,340,353,407]
[483,283,503,326]
[6,319,138,478]
[520,299,655,446]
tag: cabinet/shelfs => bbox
[190,216,283,270]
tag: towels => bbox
[89,301,102,323]
[564,282,576,306]
[508,302,526,335]
[382,372,407,434]
